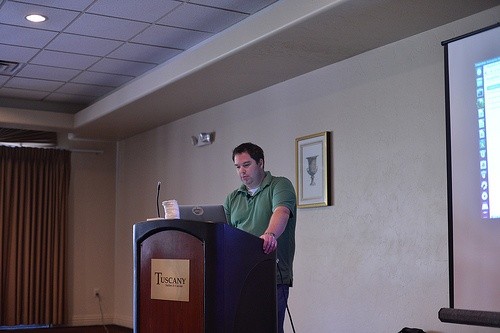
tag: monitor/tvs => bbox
[178,204,227,223]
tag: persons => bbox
[221,143,297,333]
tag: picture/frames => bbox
[295,130,330,208]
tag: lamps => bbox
[191,133,211,147]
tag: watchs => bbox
[263,232,278,240]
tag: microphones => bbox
[156,182,161,218]
